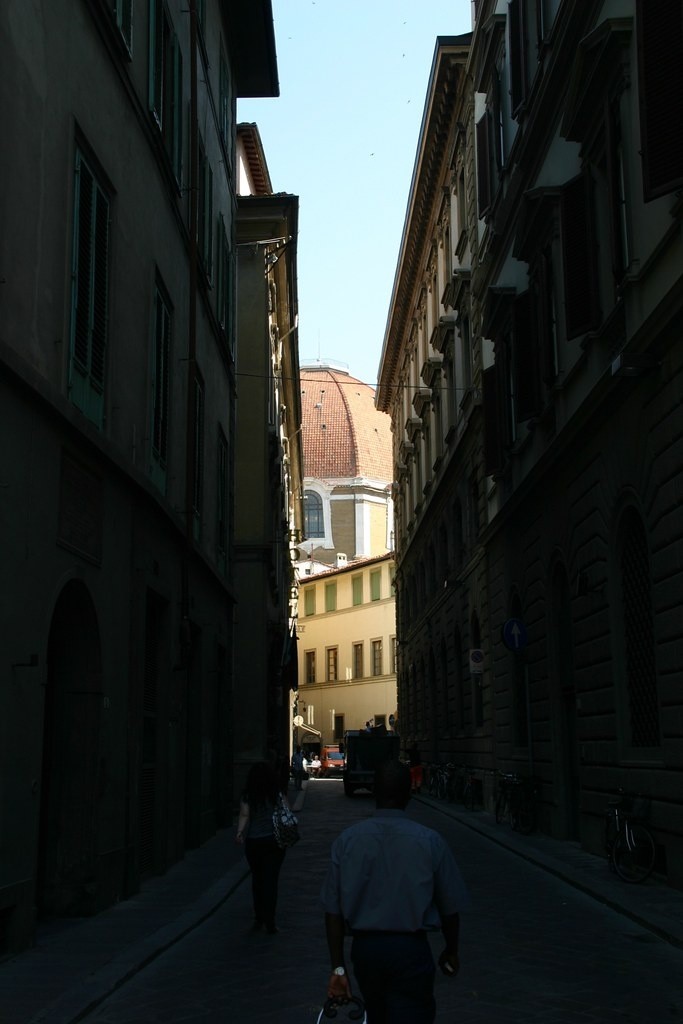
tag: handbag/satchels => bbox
[272,791,300,848]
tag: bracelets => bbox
[237,830,243,833]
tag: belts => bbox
[353,928,428,938]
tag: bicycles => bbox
[601,783,656,884]
[424,759,456,804]
[460,761,475,811]
[495,769,522,831]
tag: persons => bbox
[310,755,322,778]
[292,745,305,791]
[317,766,469,1024]
[235,764,290,937]
[409,742,424,795]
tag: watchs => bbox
[330,966,348,977]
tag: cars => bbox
[319,748,345,778]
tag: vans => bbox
[344,737,400,797]
